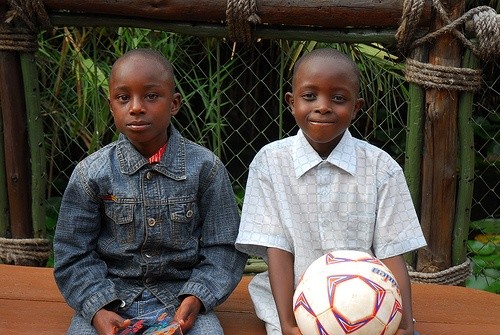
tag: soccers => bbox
[292,250,403,335]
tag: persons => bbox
[234,48,428,335]
[53,48,248,335]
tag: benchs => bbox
[0,263,500,335]
